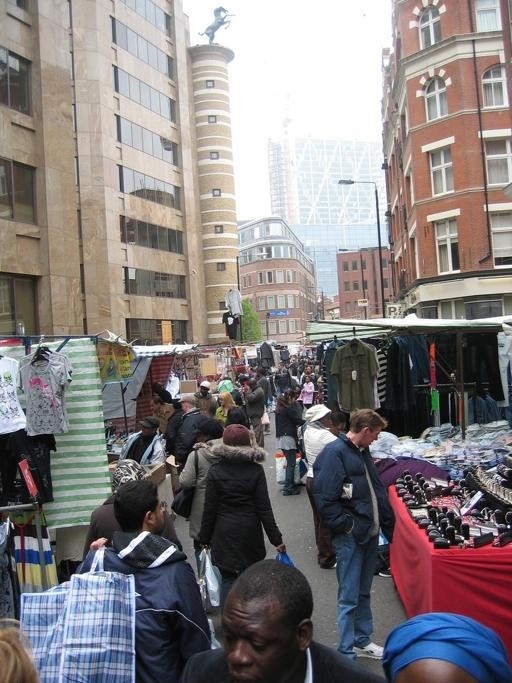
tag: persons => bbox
[1,629,41,683]
[310,407,397,659]
[178,557,387,681]
[79,338,408,589]
[72,477,214,682]
[194,423,289,615]
[376,609,510,682]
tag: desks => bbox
[385,481,510,662]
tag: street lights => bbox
[339,247,368,319]
[337,178,386,318]
[236,252,270,345]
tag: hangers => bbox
[32,335,52,366]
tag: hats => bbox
[177,395,196,404]
[305,403,332,422]
[200,380,210,391]
[138,416,161,428]
[223,424,250,445]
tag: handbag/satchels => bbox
[171,488,194,518]
[275,454,302,483]
[199,546,221,612]
[19,544,136,682]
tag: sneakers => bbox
[352,642,384,660]
[283,485,300,495]
[379,567,391,578]
